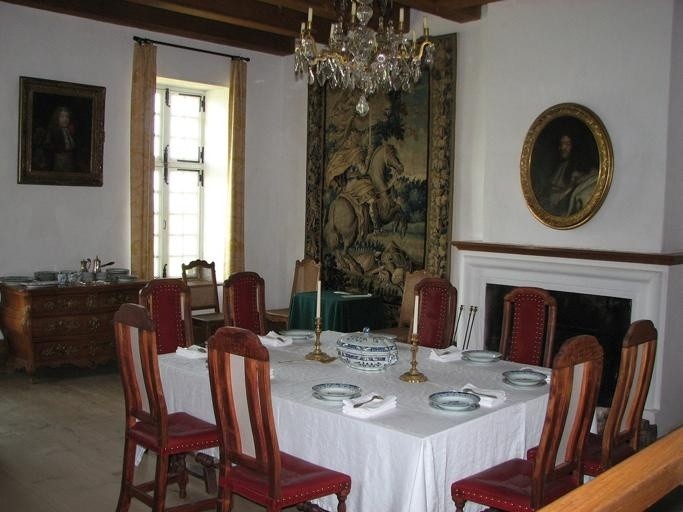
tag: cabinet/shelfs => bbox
[1,275,187,388]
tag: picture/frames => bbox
[16,74,107,192]
[517,99,619,230]
[299,30,460,331]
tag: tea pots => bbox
[91,256,102,275]
[78,257,90,272]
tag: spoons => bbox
[461,387,499,399]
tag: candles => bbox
[315,281,323,322]
[412,288,419,336]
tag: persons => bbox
[547,134,584,217]
[327,128,389,237]
[47,106,81,172]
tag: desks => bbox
[287,287,378,334]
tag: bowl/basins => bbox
[57,271,105,283]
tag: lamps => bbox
[287,0,440,122]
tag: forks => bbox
[353,394,387,409]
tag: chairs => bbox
[179,259,230,350]
[366,268,442,344]
[111,299,229,512]
[255,257,324,334]
[200,319,355,512]
[138,273,194,356]
[447,329,606,511]
[407,272,462,350]
[527,316,659,480]
[497,285,558,371]
[219,268,269,338]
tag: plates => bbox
[462,349,503,365]
[311,381,362,405]
[427,391,480,414]
[502,371,548,388]
[278,329,315,340]
[104,268,137,282]
